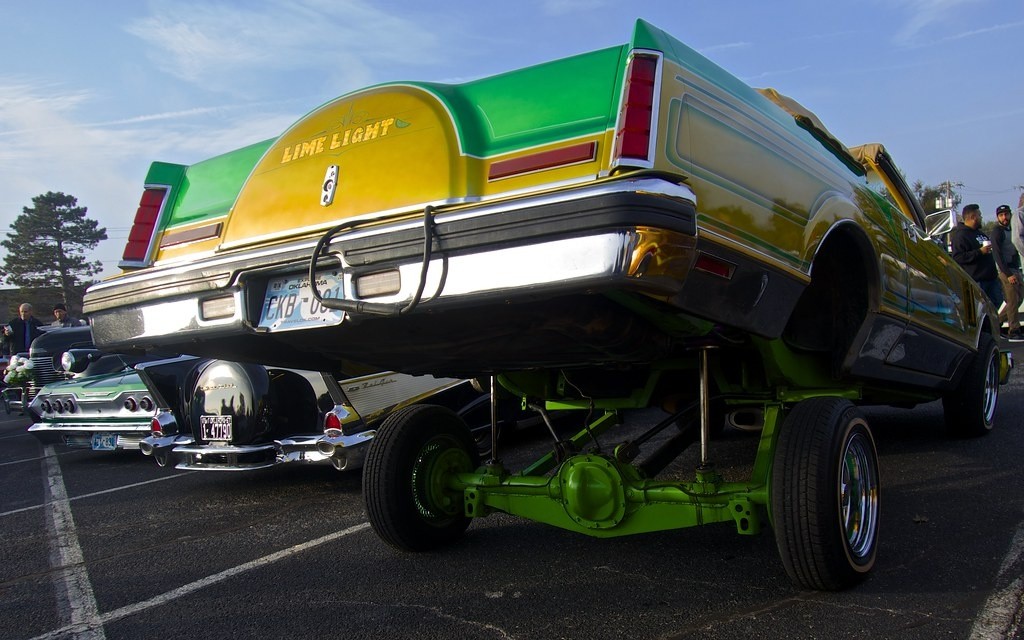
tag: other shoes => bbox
[1008,334,1024,342]
[17,412,23,416]
[1000,333,1007,339]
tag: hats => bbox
[996,205,1011,215]
[52,303,67,314]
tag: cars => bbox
[2,16,1016,595]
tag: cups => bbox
[983,241,991,253]
[4,325,13,334]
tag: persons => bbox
[949,203,1004,309]
[1011,193,1024,274]
[3,303,46,355]
[991,204,1024,343]
[51,302,82,327]
